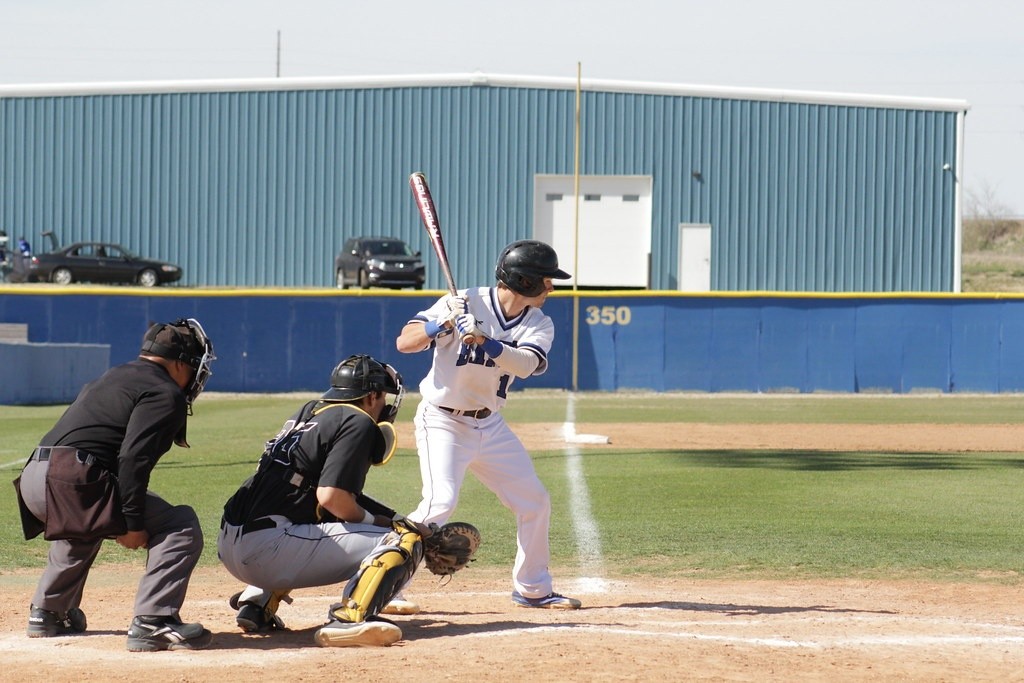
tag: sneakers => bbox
[379,592,421,615]
[26,603,87,638]
[314,618,402,648]
[235,602,286,635]
[125,615,213,651]
[511,587,581,610]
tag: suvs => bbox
[336,237,426,290]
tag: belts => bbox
[220,516,277,537]
[32,448,88,461]
[439,405,491,419]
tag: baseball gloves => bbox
[420,522,481,588]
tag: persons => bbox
[380,240,581,614]
[13,318,213,651]
[19,237,30,258]
[216,356,480,647]
[0,230,9,282]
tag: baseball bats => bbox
[408,171,477,346]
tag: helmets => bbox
[495,240,572,297]
[316,355,400,401]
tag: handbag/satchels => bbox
[12,448,45,541]
[44,446,129,541]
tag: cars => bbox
[28,231,181,287]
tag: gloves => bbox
[435,295,469,337]
[455,313,483,340]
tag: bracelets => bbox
[478,336,504,359]
[424,318,446,340]
